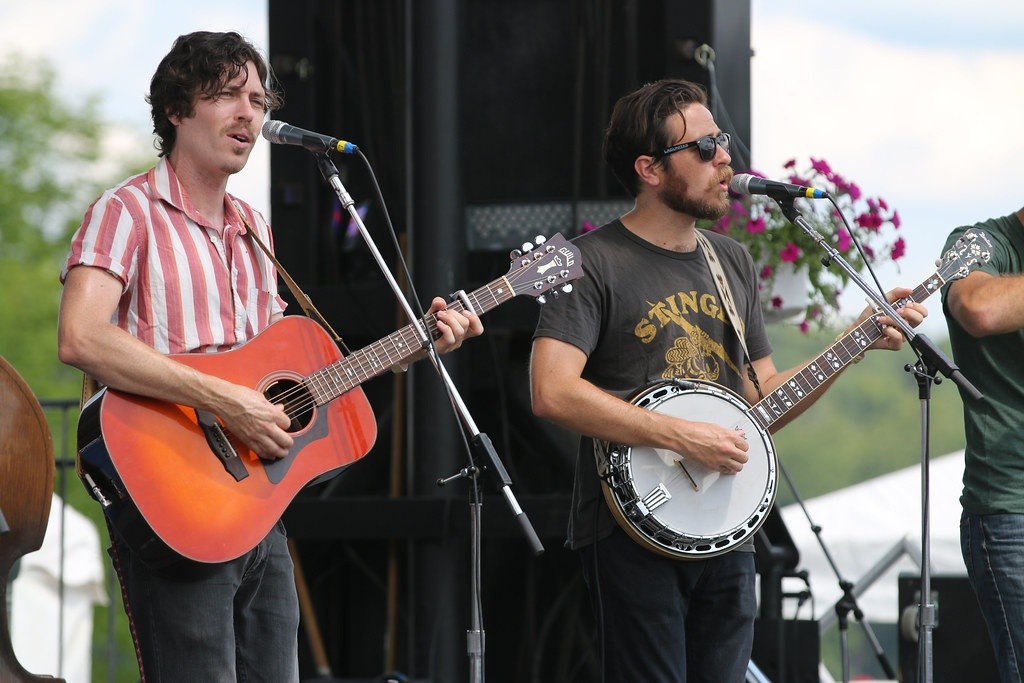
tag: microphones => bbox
[262,120,337,150]
[730,173,804,198]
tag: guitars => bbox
[599,226,997,563]
[75,231,585,585]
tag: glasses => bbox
[649,133,731,163]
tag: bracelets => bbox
[390,361,409,374]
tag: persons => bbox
[526,78,928,683]
[940,209,1023,683]
[56,31,485,681]
